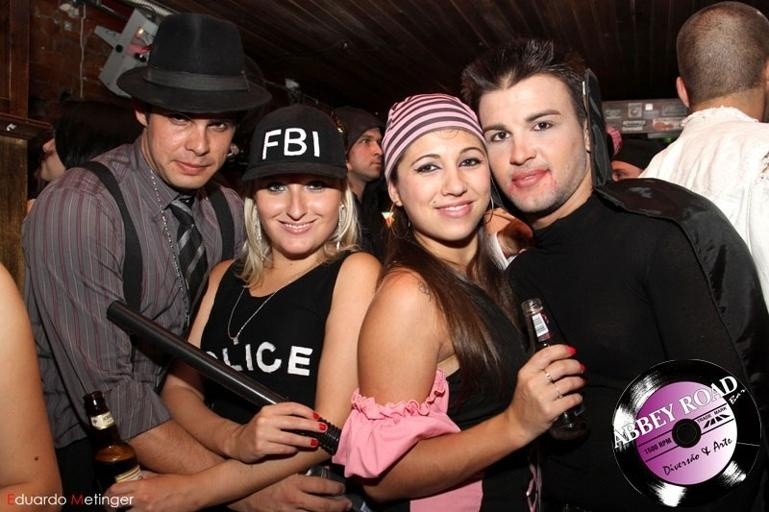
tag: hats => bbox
[55,108,140,171]
[116,11,273,116]
[331,104,383,155]
[382,92,488,184]
[241,101,347,182]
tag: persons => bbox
[0,0,768,511]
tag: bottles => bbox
[82,389,143,510]
[520,296,591,439]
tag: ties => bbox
[168,195,208,314]
